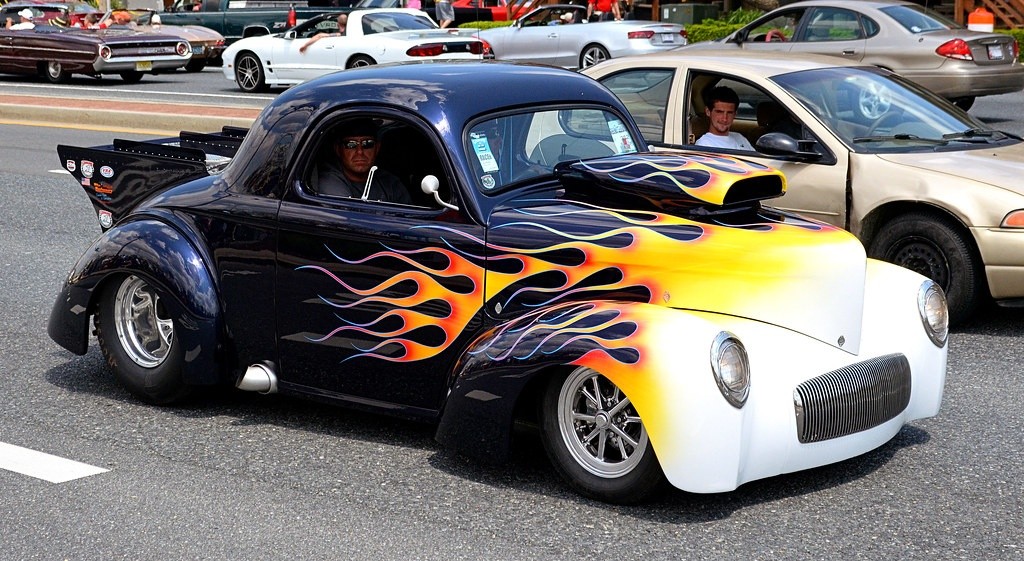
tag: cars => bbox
[712,0,1024,114]
[523,42,1024,329]
[0,0,543,84]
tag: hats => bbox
[18,9,33,21]
[327,116,384,137]
[560,12,574,22]
[337,14,347,23]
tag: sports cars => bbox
[472,4,688,83]
[220,7,493,94]
[47,56,951,504]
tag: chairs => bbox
[755,101,800,140]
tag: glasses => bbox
[339,139,378,149]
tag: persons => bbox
[151,14,161,25]
[695,86,756,151]
[81,14,114,29]
[434,0,460,29]
[560,12,573,25]
[319,115,411,204]
[192,0,202,12]
[587,0,621,21]
[116,12,137,27]
[790,10,804,31]
[6,9,36,30]
[300,14,348,53]
[48,16,66,28]
